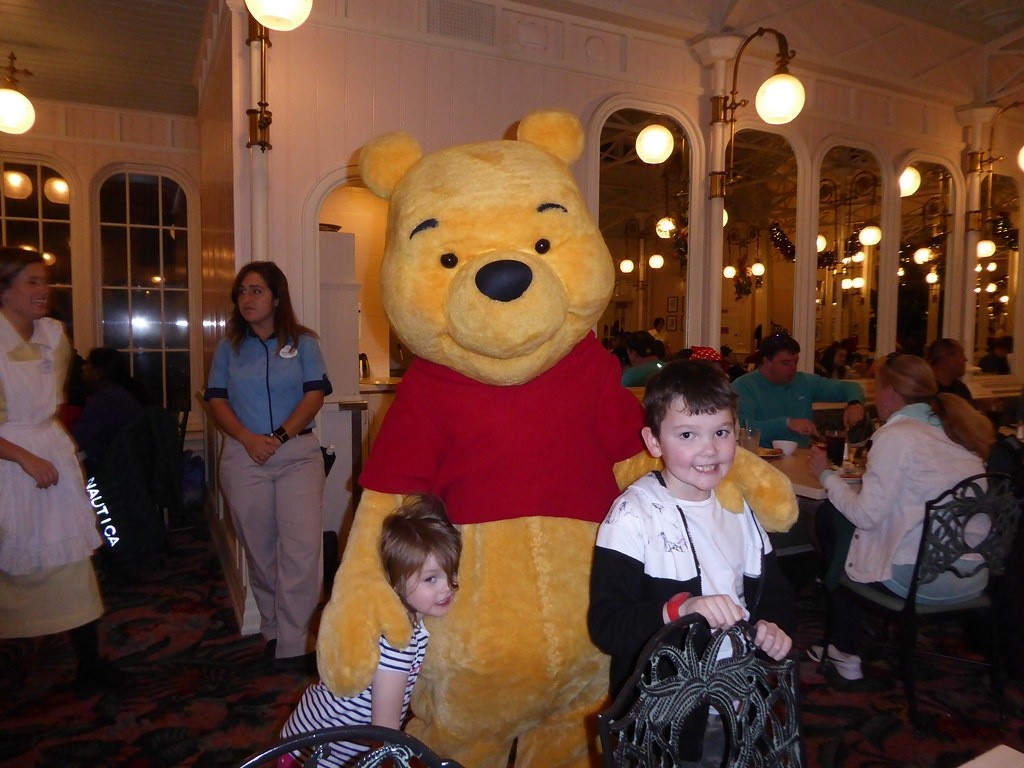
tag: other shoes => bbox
[74,656,126,690]
[753,658,796,672]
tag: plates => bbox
[757,446,784,456]
[841,477,863,484]
[807,438,827,448]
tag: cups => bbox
[825,430,847,468]
[738,428,761,454]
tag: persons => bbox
[585,358,807,768]
[273,494,462,768]
[1,243,333,722]
[675,330,1000,680]
[607,316,1016,388]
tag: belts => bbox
[294,428,312,437]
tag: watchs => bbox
[273,426,289,444]
[667,592,694,625]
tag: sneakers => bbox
[806,643,863,680]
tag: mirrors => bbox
[599,108,691,389]
[720,127,797,383]
[974,174,1021,375]
[813,144,884,377]
[896,162,955,358]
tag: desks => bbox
[812,379,876,410]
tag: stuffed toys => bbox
[315,107,798,768]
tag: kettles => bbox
[358,352,370,381]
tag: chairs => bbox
[237,725,464,768]
[816,430,1024,741]
[595,613,807,768]
[166,401,190,533]
[98,415,169,598]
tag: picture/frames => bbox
[667,297,678,312]
[666,315,677,331]
[816,298,823,342]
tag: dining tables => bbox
[764,444,865,618]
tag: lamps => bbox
[983,101,1024,222]
[817,166,948,296]
[245,0,313,153]
[722,209,765,288]
[721,27,807,187]
[619,117,691,275]
[0,51,36,135]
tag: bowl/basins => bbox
[772,440,797,456]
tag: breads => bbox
[767,448,784,455]
[840,474,863,477]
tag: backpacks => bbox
[986,432,1024,499]
